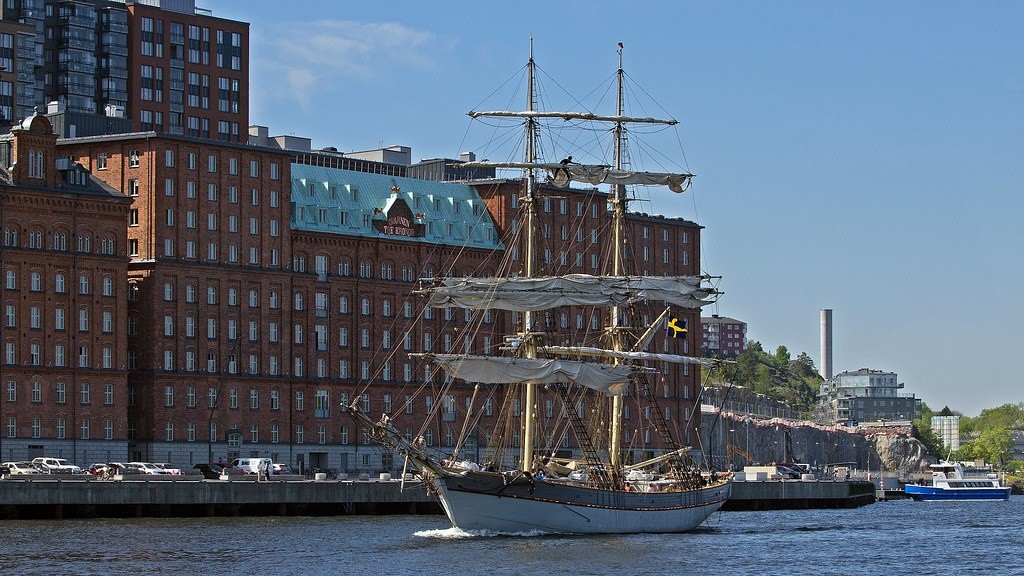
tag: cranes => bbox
[725,442,754,469]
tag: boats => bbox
[905,450,1013,500]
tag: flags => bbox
[666,316,688,339]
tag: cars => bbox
[274,463,291,474]
[193,463,230,479]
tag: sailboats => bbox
[340,34,735,533]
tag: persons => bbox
[625,481,634,491]
[257,461,270,481]
[218,456,227,463]
[536,471,546,481]
[707,470,718,486]
[487,461,495,472]
[646,482,658,492]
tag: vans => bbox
[771,463,811,479]
[231,458,276,478]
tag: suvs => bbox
[89,461,185,476]
[0,458,82,475]
